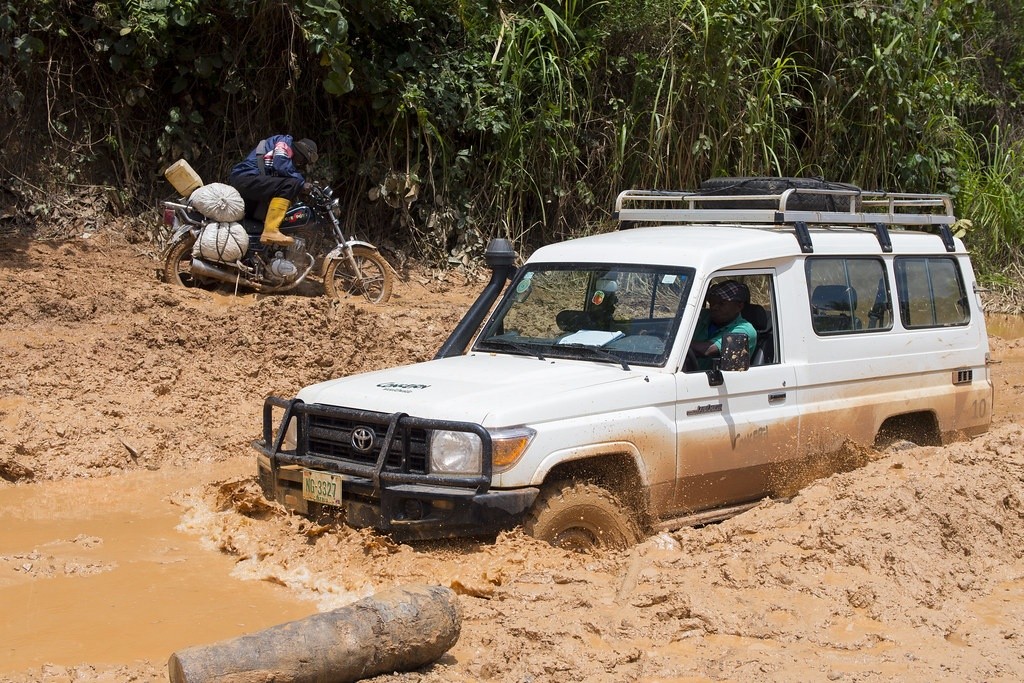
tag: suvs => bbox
[262,174,996,558]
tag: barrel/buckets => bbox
[165,159,204,199]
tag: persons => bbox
[229,135,318,293]
[638,279,756,369]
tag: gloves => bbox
[301,182,313,194]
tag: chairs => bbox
[810,286,862,331]
[742,303,771,365]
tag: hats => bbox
[706,280,752,303]
[293,138,318,167]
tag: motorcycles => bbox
[158,180,394,307]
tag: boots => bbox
[259,197,296,246]
